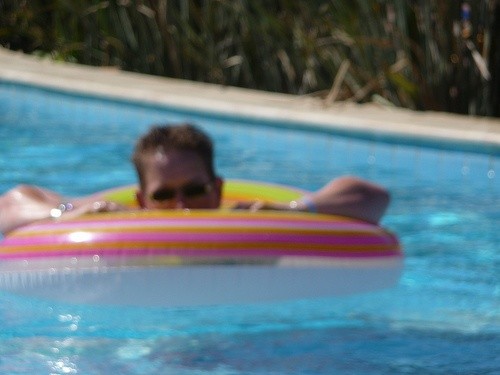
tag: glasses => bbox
[141,181,218,203]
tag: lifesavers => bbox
[0,180,403,306]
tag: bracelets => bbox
[48,203,76,221]
[301,197,320,214]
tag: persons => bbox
[0,124,391,234]
